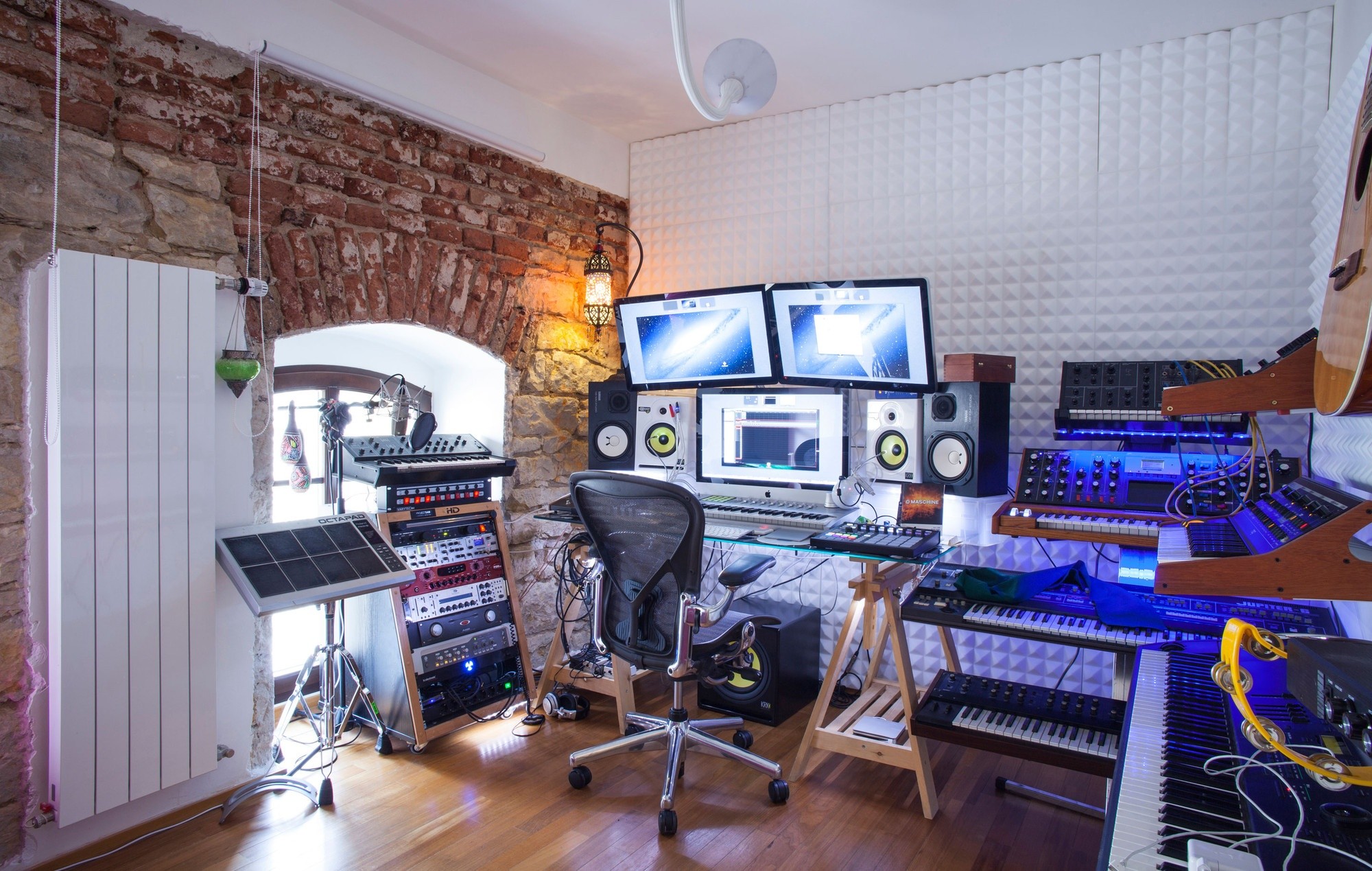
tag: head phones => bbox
[542,691,591,721]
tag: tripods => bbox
[215,511,395,806]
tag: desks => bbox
[533,507,965,820]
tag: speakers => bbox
[587,381,638,471]
[635,396,695,481]
[865,398,923,485]
[921,381,1012,497]
[695,597,821,727]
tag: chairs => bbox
[566,472,789,837]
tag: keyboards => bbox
[704,524,754,540]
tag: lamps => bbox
[582,221,644,381]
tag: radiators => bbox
[23,247,269,823]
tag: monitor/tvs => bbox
[612,284,778,392]
[765,276,939,394]
[695,388,850,490]
